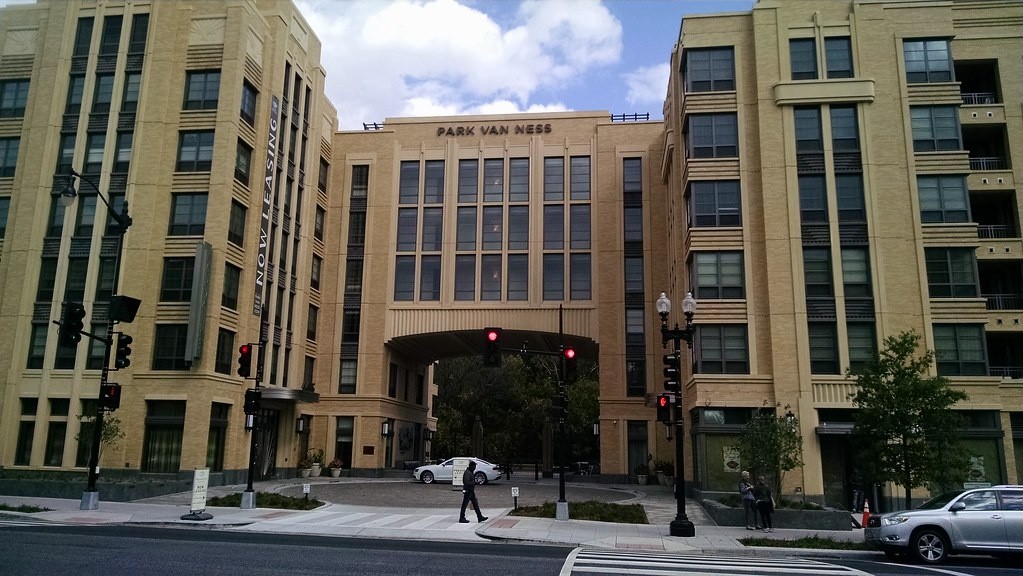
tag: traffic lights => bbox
[115,334,133,369]
[661,349,681,392]
[244,391,262,414]
[237,343,250,377]
[103,385,122,408]
[656,394,672,421]
[561,348,578,383]
[482,327,504,367]
[59,302,86,349]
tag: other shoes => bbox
[769,528,773,533]
[459,517,470,523]
[746,526,754,530]
[478,516,488,523]
[756,526,763,529]
[763,529,768,533]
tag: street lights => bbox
[656,291,697,537]
[58,164,133,491]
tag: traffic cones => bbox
[861,499,872,528]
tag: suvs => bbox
[864,484,1023,565]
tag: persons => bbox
[739,471,763,530]
[752,476,774,533]
[459,461,489,524]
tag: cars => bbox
[414,457,502,485]
